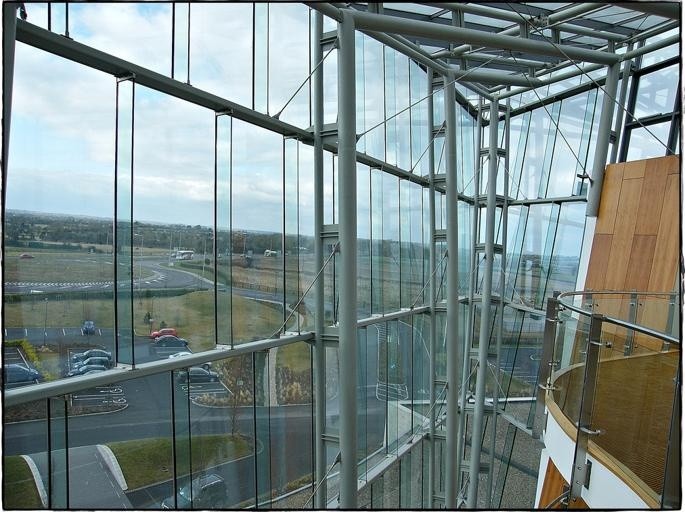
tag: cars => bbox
[167,350,222,382]
[63,346,114,379]
[216,251,257,260]
[18,252,34,259]
[82,318,95,334]
[150,328,190,346]
[171,252,176,258]
[4,360,45,383]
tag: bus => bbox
[175,250,195,260]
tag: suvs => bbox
[160,472,228,509]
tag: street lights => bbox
[134,232,145,291]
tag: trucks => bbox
[264,249,277,257]
